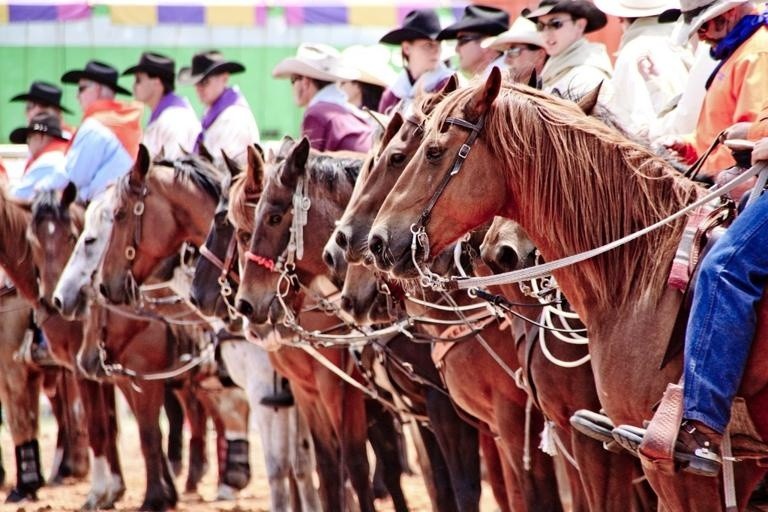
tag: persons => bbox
[176,47,262,181]
[258,40,382,408]
[329,36,389,145]
[479,7,551,102]
[570,0,766,440]
[393,4,510,122]
[380,10,452,115]
[31,57,142,203]
[524,0,614,127]
[590,0,714,170]
[613,0,768,476]
[0,112,71,212]
[6,79,73,129]
[120,51,203,168]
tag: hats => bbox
[593,0,681,18]
[122,52,175,77]
[677,0,749,45]
[59,61,132,97]
[521,1,607,34]
[435,6,508,42]
[480,16,554,56]
[318,44,399,86]
[177,50,245,88]
[9,83,74,116]
[378,8,442,44]
[271,42,342,82]
[9,111,73,142]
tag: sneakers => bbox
[13,338,49,362]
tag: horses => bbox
[0,66,763,512]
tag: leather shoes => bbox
[258,385,294,408]
[611,418,723,477]
[569,408,616,444]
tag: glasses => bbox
[455,34,484,46]
[135,76,141,84]
[504,47,528,59]
[25,133,37,143]
[78,84,92,95]
[200,76,208,86]
[290,75,303,85]
[696,22,710,34]
[23,102,37,109]
[535,18,573,31]
